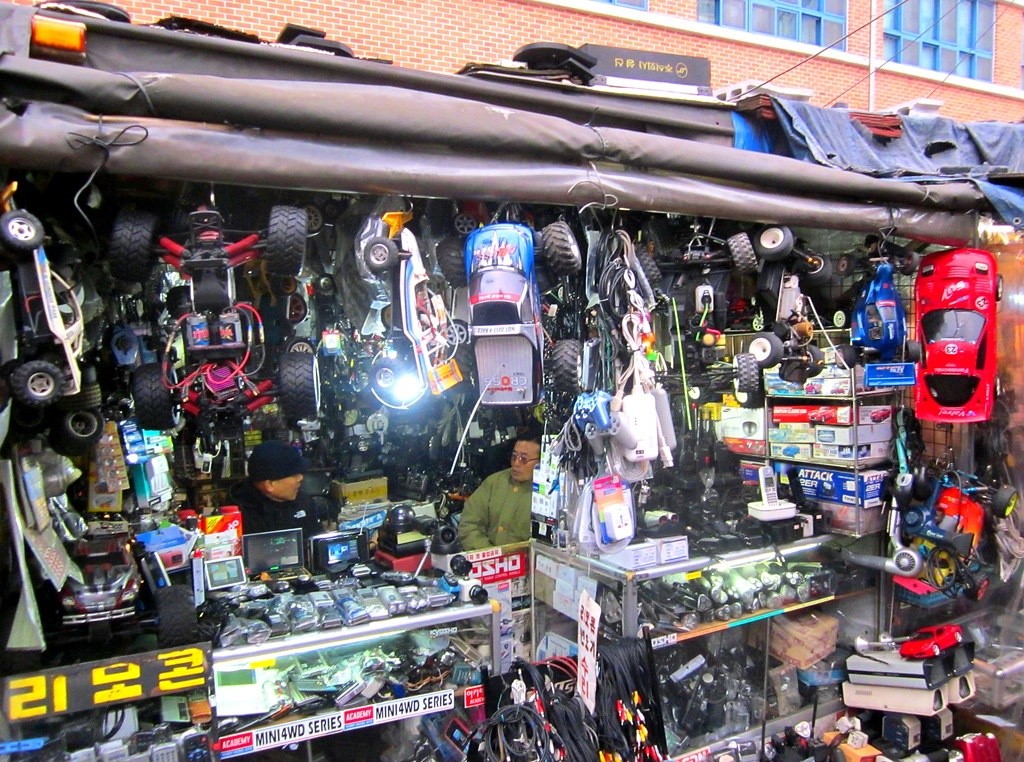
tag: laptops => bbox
[241,527,311,581]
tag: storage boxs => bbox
[703,345,897,534]
[331,474,388,507]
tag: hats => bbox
[247,440,311,483]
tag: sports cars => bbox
[914,246,1005,423]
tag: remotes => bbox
[150,742,179,762]
[184,734,211,762]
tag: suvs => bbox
[0,175,1024,652]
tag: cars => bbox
[899,623,965,659]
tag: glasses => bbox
[511,452,540,465]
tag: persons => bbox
[212,439,329,552]
[459,430,543,551]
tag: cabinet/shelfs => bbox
[701,368,893,650]
[210,596,501,762]
[528,531,889,750]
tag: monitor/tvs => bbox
[309,529,370,576]
[204,555,247,590]
